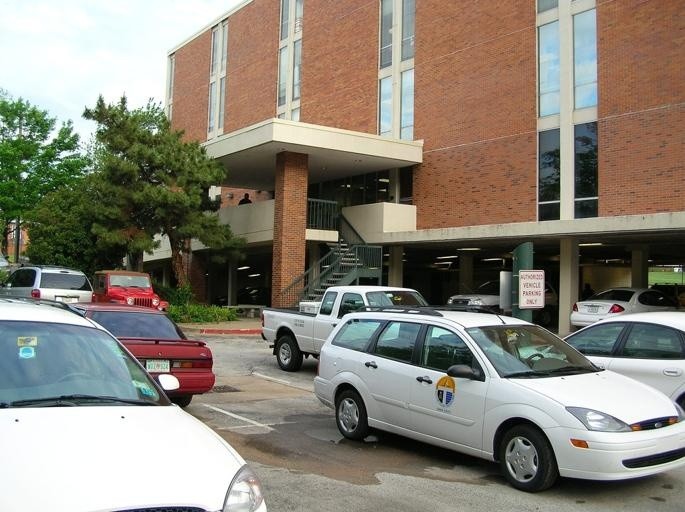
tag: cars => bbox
[518,311,684,406]
[315,304,684,493]
[570,286,683,328]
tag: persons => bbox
[237,193,252,205]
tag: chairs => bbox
[472,332,507,375]
[637,333,660,358]
[427,336,454,370]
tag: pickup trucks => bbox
[260,286,428,371]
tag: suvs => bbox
[447,278,559,327]
